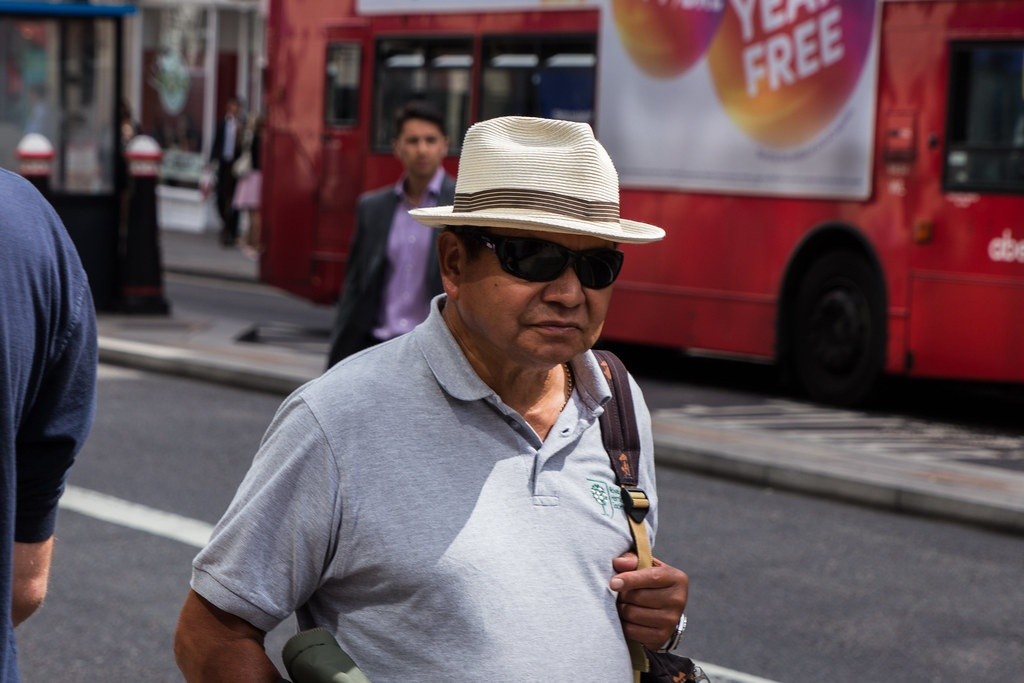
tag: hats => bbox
[408,115,665,243]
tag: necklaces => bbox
[559,362,574,412]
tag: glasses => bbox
[458,230,625,289]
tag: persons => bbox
[323,100,457,372]
[173,116,689,683]
[0,90,261,259]
[0,167,99,683]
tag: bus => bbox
[249,0,1023,430]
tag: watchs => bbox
[658,613,688,653]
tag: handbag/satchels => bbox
[234,170,262,206]
